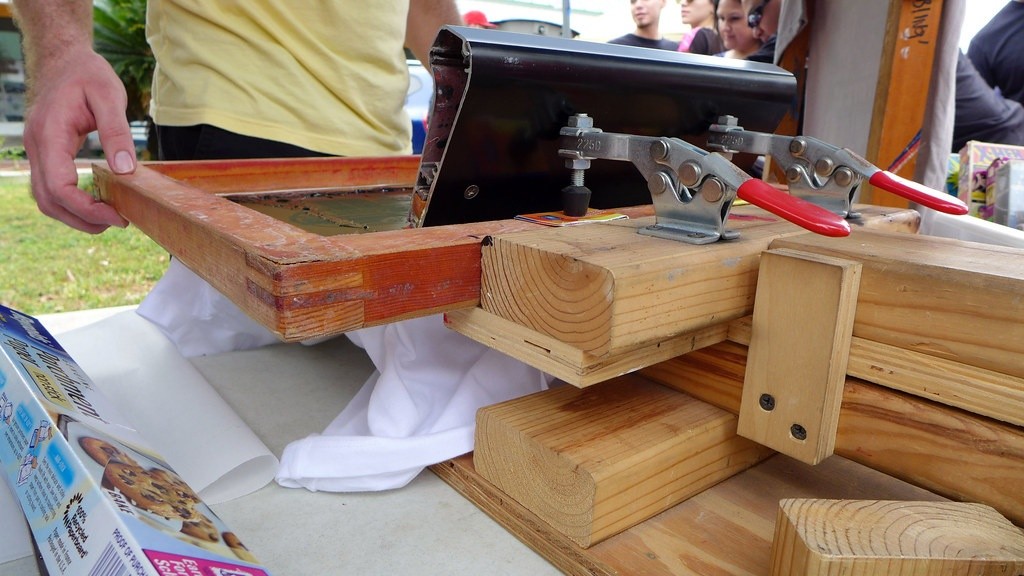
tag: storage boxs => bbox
[0,303,268,576]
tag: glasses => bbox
[747,0,770,28]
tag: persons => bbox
[460,11,498,30]
[951,0,1024,157]
[10,0,465,235]
[605,0,782,68]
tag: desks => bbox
[177,340,571,575]
